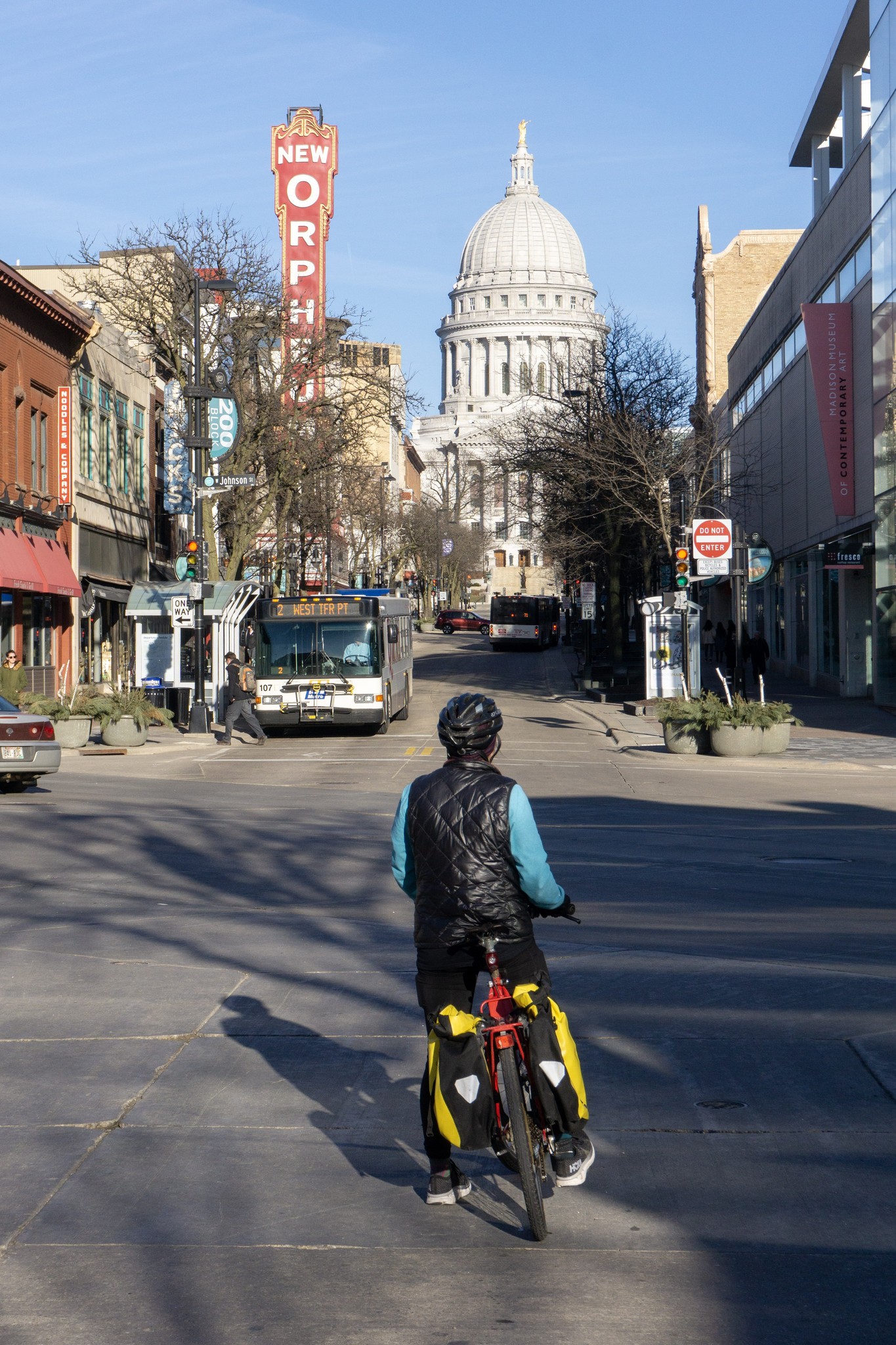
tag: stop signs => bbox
[692,519,733,559]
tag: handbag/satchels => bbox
[424,1002,494,1150]
[511,975,588,1137]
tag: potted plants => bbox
[17,672,176,746]
[650,692,804,757]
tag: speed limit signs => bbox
[582,602,595,621]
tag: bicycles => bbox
[473,904,582,1243]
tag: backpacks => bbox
[230,662,255,692]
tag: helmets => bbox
[437,693,502,748]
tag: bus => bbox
[255,589,413,734]
[490,591,561,651]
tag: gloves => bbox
[540,894,572,918]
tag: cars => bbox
[0,695,61,784]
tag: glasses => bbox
[9,656,16,658]
[226,659,228,663]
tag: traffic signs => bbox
[562,596,570,609]
[170,596,194,629]
[576,597,582,608]
[440,591,447,601]
[467,588,471,593]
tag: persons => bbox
[412,608,418,618]
[701,620,716,663]
[713,620,769,684]
[456,368,464,385]
[464,614,469,618]
[446,384,454,398]
[244,625,256,666]
[216,651,267,745]
[458,383,466,396]
[519,572,526,588]
[518,119,531,142]
[390,692,596,1205]
[0,650,28,708]
[342,635,370,665]
[434,603,437,616]
[81,646,94,675]
[205,640,212,659]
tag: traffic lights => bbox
[185,540,199,580]
[675,546,691,588]
[576,579,581,596]
[432,579,437,591]
[467,574,472,584]
[563,580,566,592]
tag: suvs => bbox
[435,609,490,635]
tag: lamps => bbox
[0,479,78,525]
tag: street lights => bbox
[564,387,593,580]
[184,278,241,731]
[436,508,457,614]
[380,476,397,589]
[260,533,322,598]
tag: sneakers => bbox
[256,735,267,745]
[425,1160,472,1206]
[550,1129,596,1189]
[217,740,231,745]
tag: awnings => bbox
[21,532,82,597]
[79,580,133,618]
[0,527,43,591]
